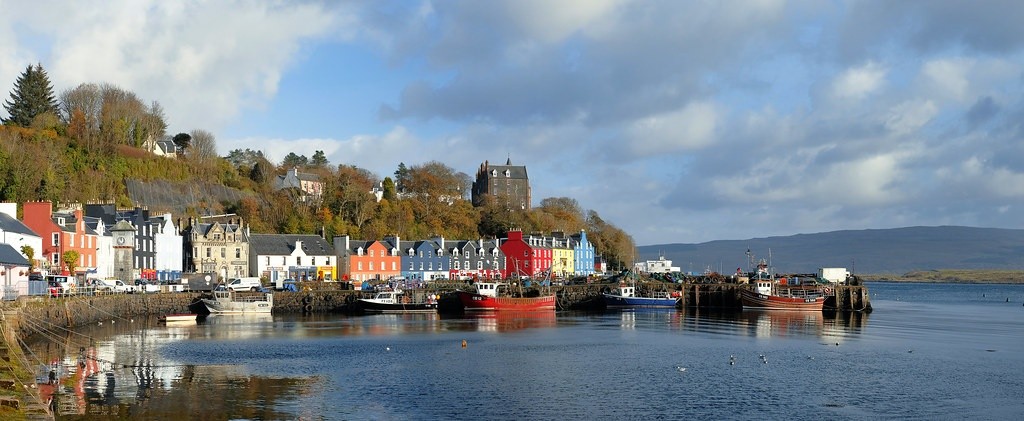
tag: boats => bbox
[735,247,835,311]
[356,280,439,315]
[455,253,557,312]
[200,278,274,313]
[599,277,683,311]
[155,313,198,322]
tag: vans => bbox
[224,276,261,293]
[42,275,161,299]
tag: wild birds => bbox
[676,367,690,372]
[729,354,737,365]
[759,354,768,364]
